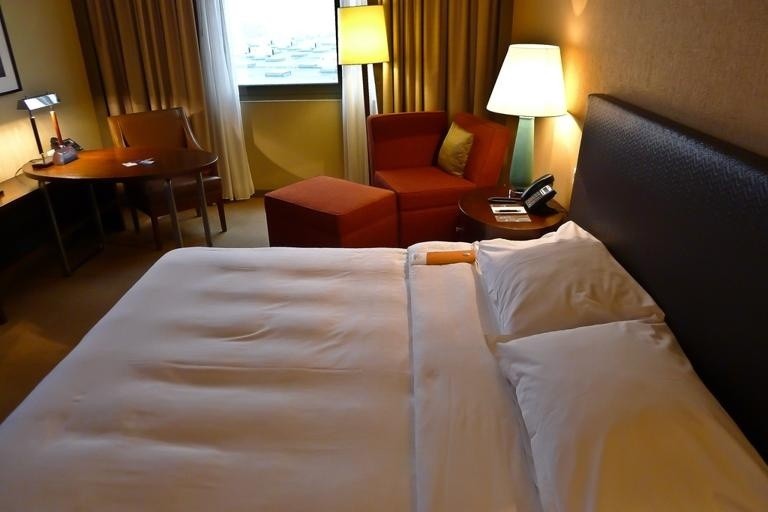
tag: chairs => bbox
[107,106,227,251]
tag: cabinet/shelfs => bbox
[0,174,127,324]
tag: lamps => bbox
[338,5,390,115]
[486,44,567,190]
[16,91,62,168]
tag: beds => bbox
[0,93,768,512]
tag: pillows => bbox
[470,221,666,334]
[484,312,768,512]
[438,121,474,175]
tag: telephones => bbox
[488,174,556,210]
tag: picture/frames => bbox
[0,9,23,96]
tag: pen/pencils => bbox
[495,210,520,213]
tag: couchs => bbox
[264,176,397,248]
[366,111,512,248]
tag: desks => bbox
[22,148,218,270]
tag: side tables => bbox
[459,186,567,240]
